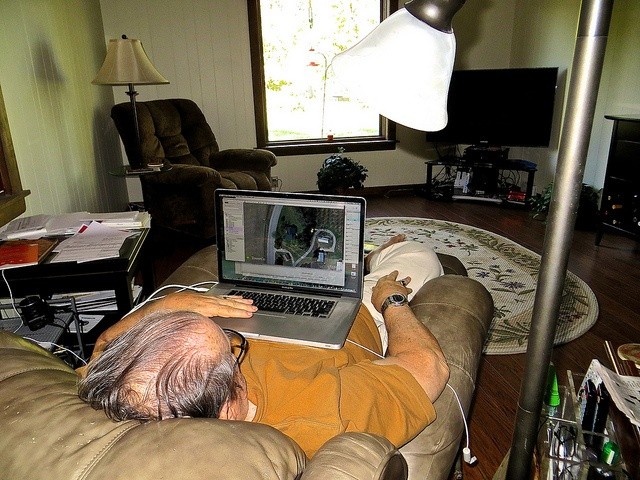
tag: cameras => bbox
[19,294,54,332]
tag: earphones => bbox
[461,446,477,465]
[201,188,367,352]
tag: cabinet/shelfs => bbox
[425,157,538,210]
[592,111,640,258]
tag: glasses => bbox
[222,328,249,370]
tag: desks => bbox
[1,216,154,315]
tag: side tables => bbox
[109,164,174,219]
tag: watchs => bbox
[380,292,412,316]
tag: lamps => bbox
[331,1,614,480]
[89,32,172,172]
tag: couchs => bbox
[1,245,495,480]
[107,98,279,234]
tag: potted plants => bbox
[318,152,368,198]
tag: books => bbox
[50,290,116,314]
[0,239,59,272]
[2,210,151,239]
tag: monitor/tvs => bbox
[420,64,560,162]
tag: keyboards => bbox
[452,189,504,209]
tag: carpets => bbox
[364,214,598,358]
